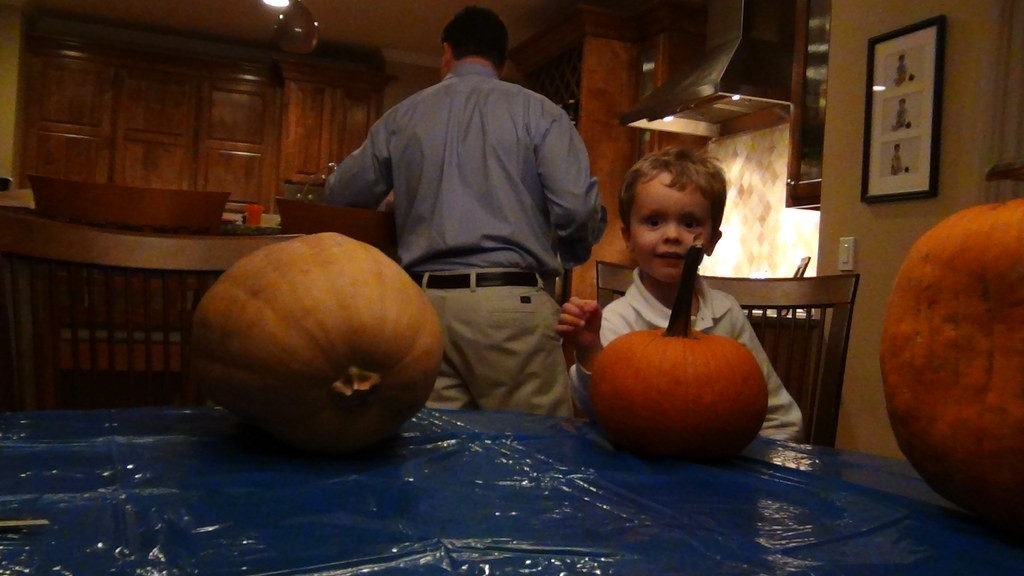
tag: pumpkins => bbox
[191,234,446,449]
[588,245,766,462]
[881,199,1023,523]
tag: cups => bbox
[246,205,263,227]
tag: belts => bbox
[408,270,538,289]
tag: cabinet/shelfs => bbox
[509,4,640,306]
[19,29,386,213]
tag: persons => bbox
[891,98,908,131]
[554,146,803,443]
[322,4,609,419]
[891,143,903,175]
[894,55,906,87]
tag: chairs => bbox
[595,260,861,449]
[0,207,305,415]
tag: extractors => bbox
[623,0,793,137]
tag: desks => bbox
[0,407,1023,575]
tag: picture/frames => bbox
[860,14,946,203]
